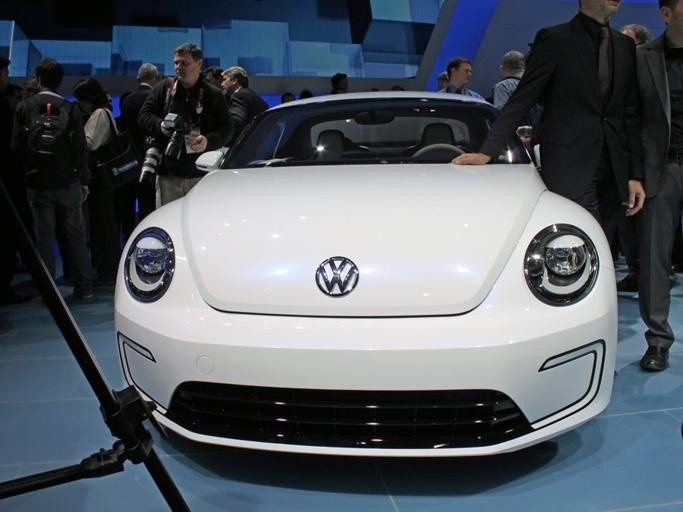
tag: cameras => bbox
[161,111,196,161]
[138,135,164,189]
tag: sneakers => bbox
[73,289,93,299]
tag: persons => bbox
[493,49,528,105]
[280,91,296,105]
[1,39,269,305]
[616,22,651,49]
[635,0,683,374]
[437,58,478,98]
[437,69,449,88]
[299,89,314,99]
[330,72,351,92]
[448,0,647,247]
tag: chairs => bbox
[310,122,456,161]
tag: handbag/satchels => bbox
[88,140,141,191]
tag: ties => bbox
[599,26,613,97]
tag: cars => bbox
[112,87,622,457]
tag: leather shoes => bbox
[639,346,669,372]
[617,275,639,292]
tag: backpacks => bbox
[21,97,76,191]
